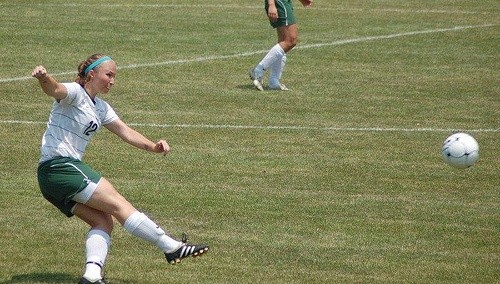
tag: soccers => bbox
[441,131,480,171]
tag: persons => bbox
[31,52,209,284]
[248,0,314,91]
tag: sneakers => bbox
[268,84,289,91]
[78,277,106,284]
[164,233,209,265]
[249,69,264,91]
